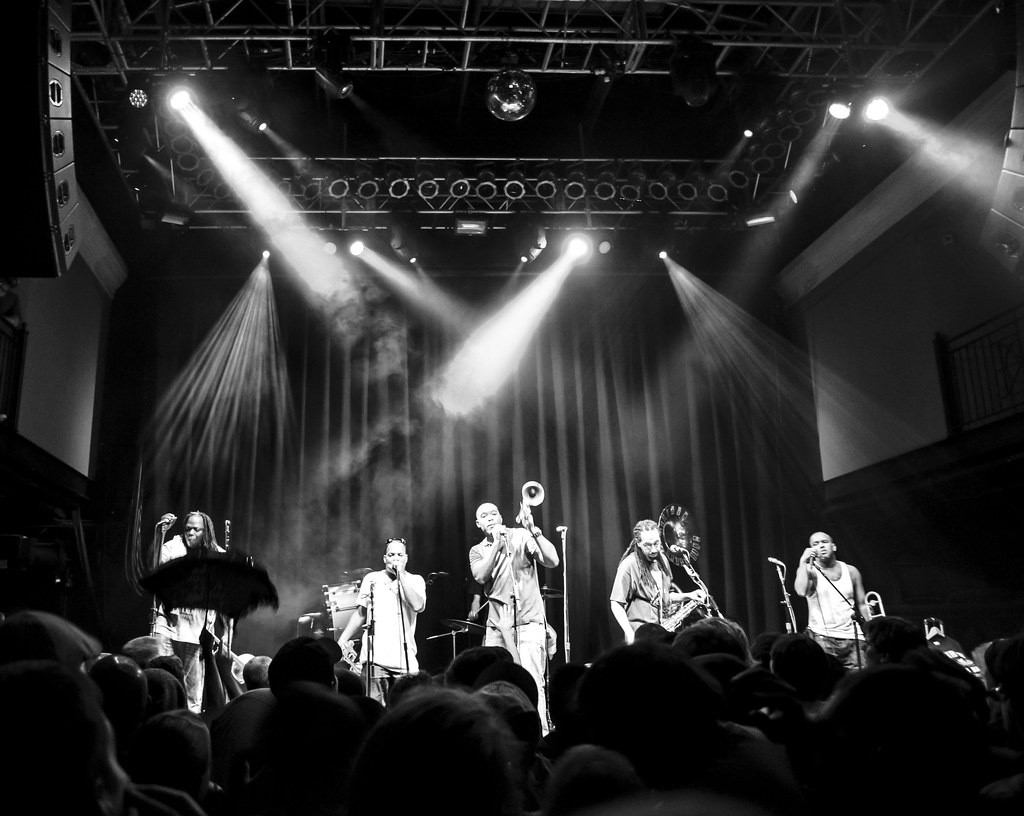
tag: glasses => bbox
[387,537,406,545]
[185,526,204,532]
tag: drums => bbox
[321,582,366,645]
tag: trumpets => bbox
[514,480,545,523]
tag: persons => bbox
[337,535,426,705]
[610,520,706,644]
[470,501,560,737]
[794,530,872,671]
[0,608,1024,816]
[152,510,227,716]
[468,578,481,620]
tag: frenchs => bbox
[656,503,699,631]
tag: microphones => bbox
[767,557,785,567]
[499,529,508,538]
[157,519,169,526]
[669,544,688,553]
[810,546,818,569]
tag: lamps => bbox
[114,72,894,263]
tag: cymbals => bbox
[539,588,570,599]
[337,567,372,579]
[439,618,485,633]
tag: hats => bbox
[269,636,341,704]
